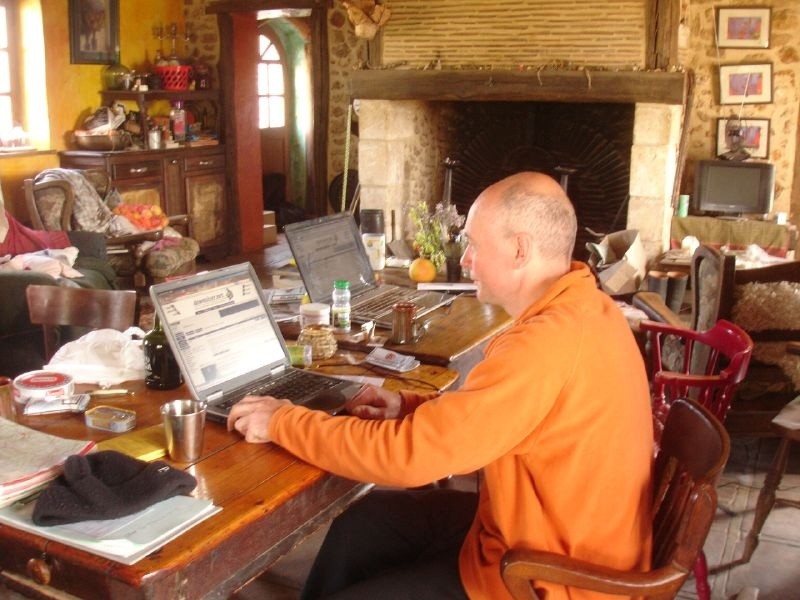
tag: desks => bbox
[0,265,516,600]
[646,248,787,274]
[670,216,790,260]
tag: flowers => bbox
[408,200,466,266]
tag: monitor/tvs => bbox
[691,158,776,222]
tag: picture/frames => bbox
[716,7,772,49]
[716,117,771,159]
[69,0,121,65]
[719,62,774,106]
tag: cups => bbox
[360,232,386,270]
[148,129,161,150]
[159,399,208,463]
[358,210,384,234]
[391,302,417,345]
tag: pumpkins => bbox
[408,255,436,282]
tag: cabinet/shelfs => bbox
[58,86,234,260]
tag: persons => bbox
[226,172,653,600]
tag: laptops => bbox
[148,261,367,425]
[281,210,457,329]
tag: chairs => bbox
[26,284,136,364]
[23,169,200,290]
[0,199,118,364]
[499,245,800,600]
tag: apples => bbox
[111,202,169,229]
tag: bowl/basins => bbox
[75,130,132,152]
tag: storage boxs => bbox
[585,228,644,296]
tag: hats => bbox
[33,450,197,527]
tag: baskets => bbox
[156,67,191,90]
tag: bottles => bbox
[331,281,352,333]
[197,69,208,89]
[143,308,184,389]
[154,49,179,66]
[170,100,187,143]
[104,45,132,90]
